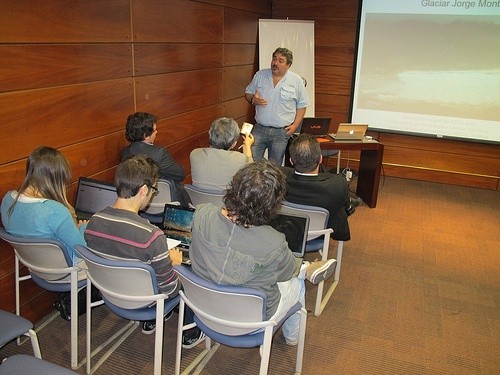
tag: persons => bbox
[283,135,362,241]
[245,48,308,166]
[189,161,338,346]
[1,146,95,322]
[190,118,254,192]
[84,156,205,350]
[119,112,192,217]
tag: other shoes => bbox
[142,310,174,334]
[181,327,207,349]
[307,259,337,284]
[346,197,362,216]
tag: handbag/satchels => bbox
[54,291,95,322]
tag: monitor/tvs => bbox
[268,212,310,258]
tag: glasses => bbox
[140,183,158,195]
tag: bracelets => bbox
[251,97,254,105]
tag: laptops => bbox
[329,123,368,139]
[162,204,195,266]
[74,176,118,224]
[292,117,331,137]
